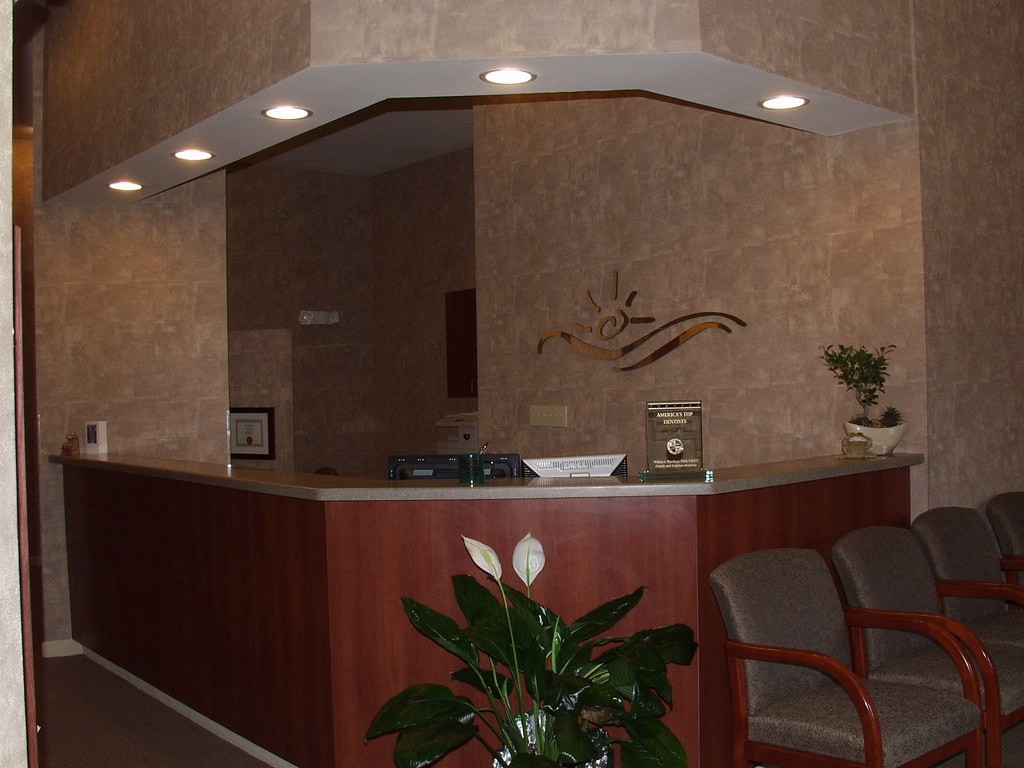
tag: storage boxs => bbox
[434,411,479,454]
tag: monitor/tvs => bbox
[521,453,627,477]
[388,454,519,480]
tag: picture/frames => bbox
[230,407,277,461]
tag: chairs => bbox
[708,546,983,768]
[829,525,1024,768]
[985,490,1024,610]
[910,506,1024,646]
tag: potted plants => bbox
[818,343,908,455]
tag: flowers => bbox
[362,533,698,768]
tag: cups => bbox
[460,453,483,483]
